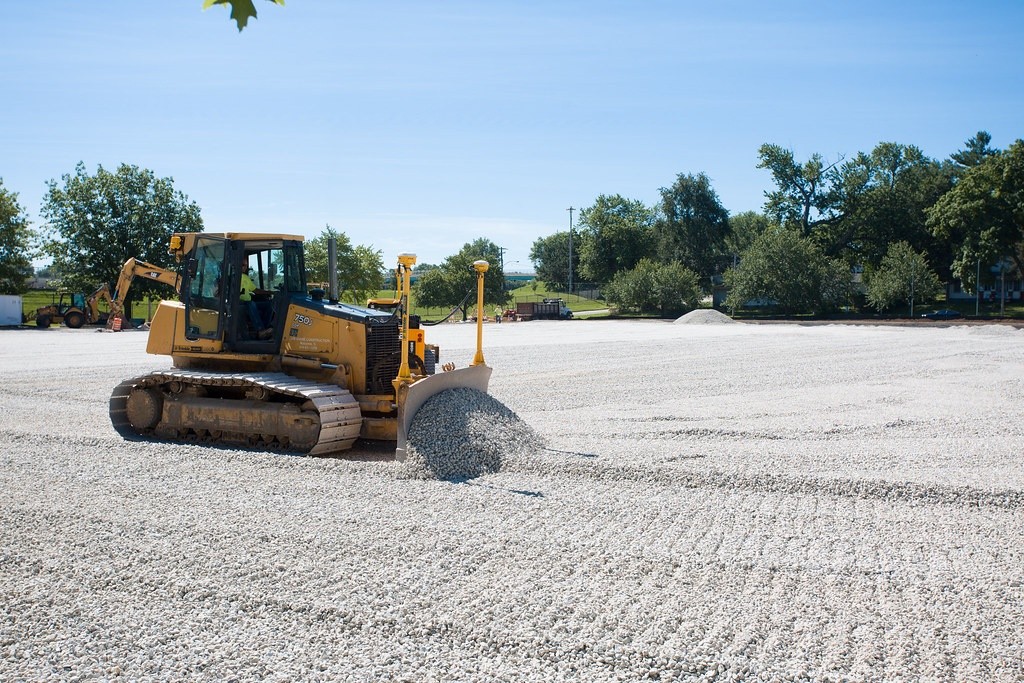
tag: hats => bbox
[497,305,501,307]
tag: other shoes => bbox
[259,327,272,337]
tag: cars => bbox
[921,308,963,321]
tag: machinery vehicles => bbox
[109,232,494,479]
[36,281,116,329]
[106,257,183,331]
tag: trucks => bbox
[514,298,574,322]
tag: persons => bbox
[213,255,272,339]
[494,305,502,324]
